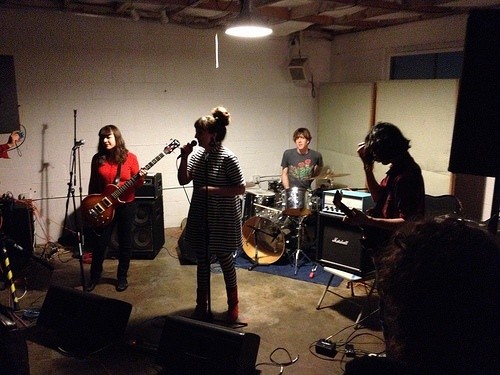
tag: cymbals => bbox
[309,173,349,179]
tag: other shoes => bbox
[86,277,99,292]
[116,277,128,291]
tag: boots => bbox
[226,285,238,321]
[192,288,208,317]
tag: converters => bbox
[315,339,337,356]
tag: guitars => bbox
[81,138,180,229]
[333,189,377,274]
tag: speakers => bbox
[0,55,20,134]
[315,212,375,275]
[448,8,500,179]
[31,283,132,359]
[157,316,260,375]
[105,195,165,259]
[287,57,311,86]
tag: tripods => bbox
[284,217,312,275]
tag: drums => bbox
[243,190,276,219]
[241,209,295,265]
[281,186,312,215]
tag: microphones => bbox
[177,140,197,159]
[272,229,281,242]
[72,139,85,150]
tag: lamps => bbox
[224,0,273,39]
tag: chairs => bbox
[316,266,383,329]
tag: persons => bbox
[343,122,425,277]
[178,107,246,324]
[342,213,500,375]
[281,128,323,190]
[84,125,149,292]
